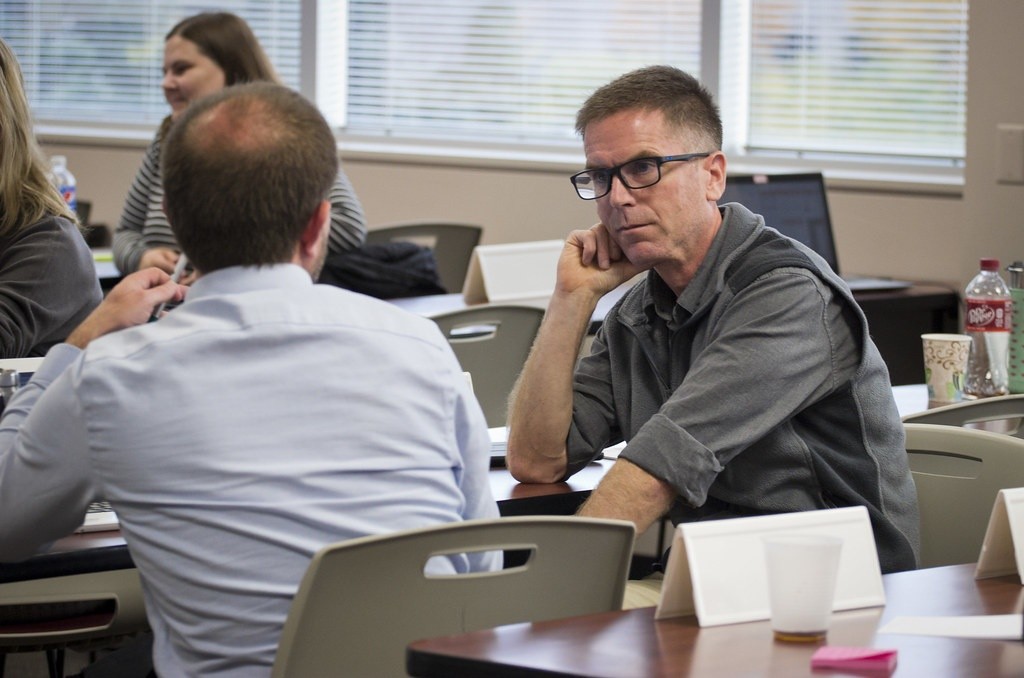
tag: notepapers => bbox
[811,645,898,670]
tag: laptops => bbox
[715,172,913,293]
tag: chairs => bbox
[0,222,1023,678]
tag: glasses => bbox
[569,152,710,201]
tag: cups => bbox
[920,334,973,404]
[762,535,842,642]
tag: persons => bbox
[0,41,103,358]
[506,65,918,612]
[113,10,366,284]
[0,79,506,678]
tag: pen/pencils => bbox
[147,251,189,322]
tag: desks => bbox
[91,247,121,292]
[382,276,961,386]
[405,556,1023,677]
[2,385,1024,586]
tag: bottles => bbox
[50,154,78,213]
[961,259,1012,396]
